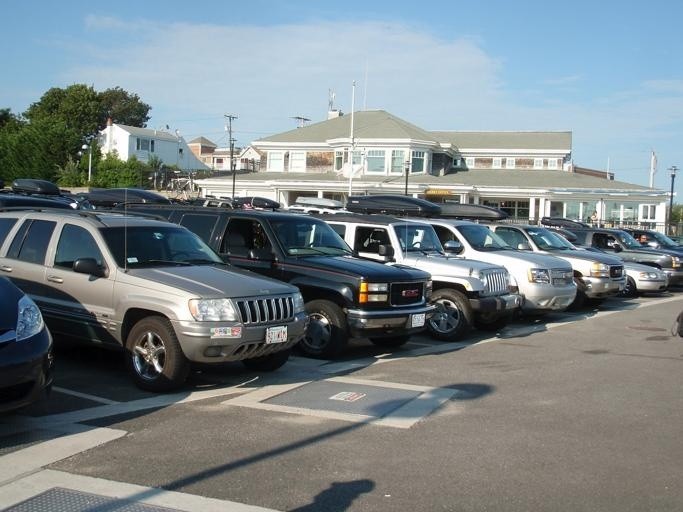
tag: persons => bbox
[591,211,599,227]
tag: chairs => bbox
[226,233,250,257]
[367,231,386,250]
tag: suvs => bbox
[131,207,435,356]
[1,208,307,391]
[299,214,523,339]
[0,180,351,217]
[344,195,578,318]
[437,202,629,307]
[540,216,681,288]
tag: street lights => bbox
[668,165,678,237]
[231,155,238,199]
[400,161,415,197]
[81,136,94,182]
[227,137,238,174]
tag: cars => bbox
[620,229,683,266]
[620,261,669,298]
[0,274,60,409]
